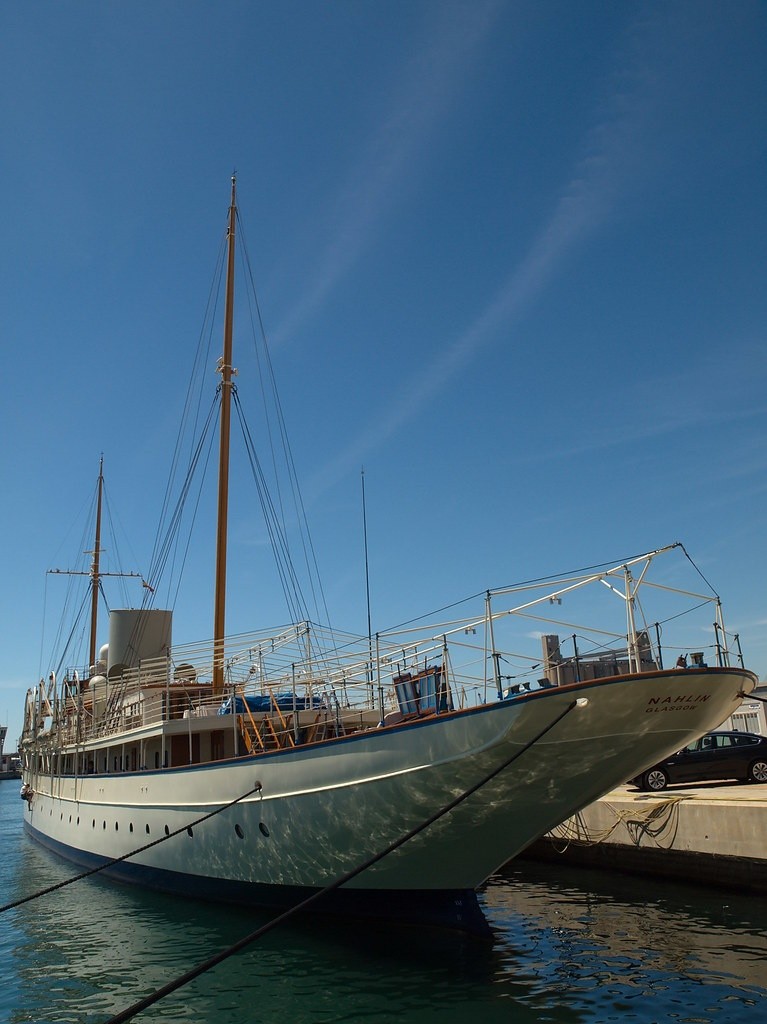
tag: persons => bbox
[20,784,34,811]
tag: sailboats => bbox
[17,163,764,916]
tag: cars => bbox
[627,730,767,792]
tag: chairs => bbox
[703,738,711,751]
